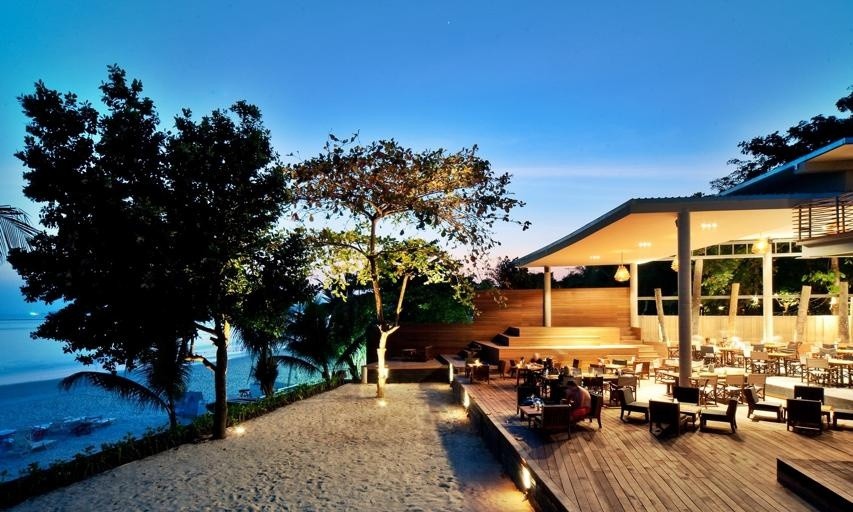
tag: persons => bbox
[518,375,544,405]
[565,380,591,424]
[704,338,711,345]
[530,352,543,366]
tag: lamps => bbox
[670,255,678,272]
[614,252,630,282]
[751,231,770,255]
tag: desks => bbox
[604,364,625,374]
[768,352,793,376]
[517,363,544,387]
[828,359,853,389]
[540,374,559,398]
[663,372,748,394]
[718,347,735,366]
[765,344,786,353]
[597,373,619,393]
[837,350,853,356]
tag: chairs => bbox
[613,359,627,367]
[648,400,688,434]
[829,344,838,348]
[573,359,579,368]
[609,377,637,406]
[589,364,605,374]
[786,399,823,435]
[509,360,516,379]
[633,361,650,380]
[822,344,830,349]
[672,386,700,406]
[698,375,718,406]
[747,374,767,401]
[723,375,744,405]
[634,363,643,388]
[583,377,603,405]
[798,352,818,382]
[465,358,479,378]
[819,348,836,355]
[833,409,853,429]
[753,345,764,352]
[750,351,775,376]
[617,387,649,423]
[699,397,738,434]
[700,346,717,366]
[572,393,603,430]
[744,386,784,422]
[535,405,572,440]
[794,385,825,405]
[787,345,797,351]
[516,386,540,416]
[806,358,837,388]
[469,364,490,385]
